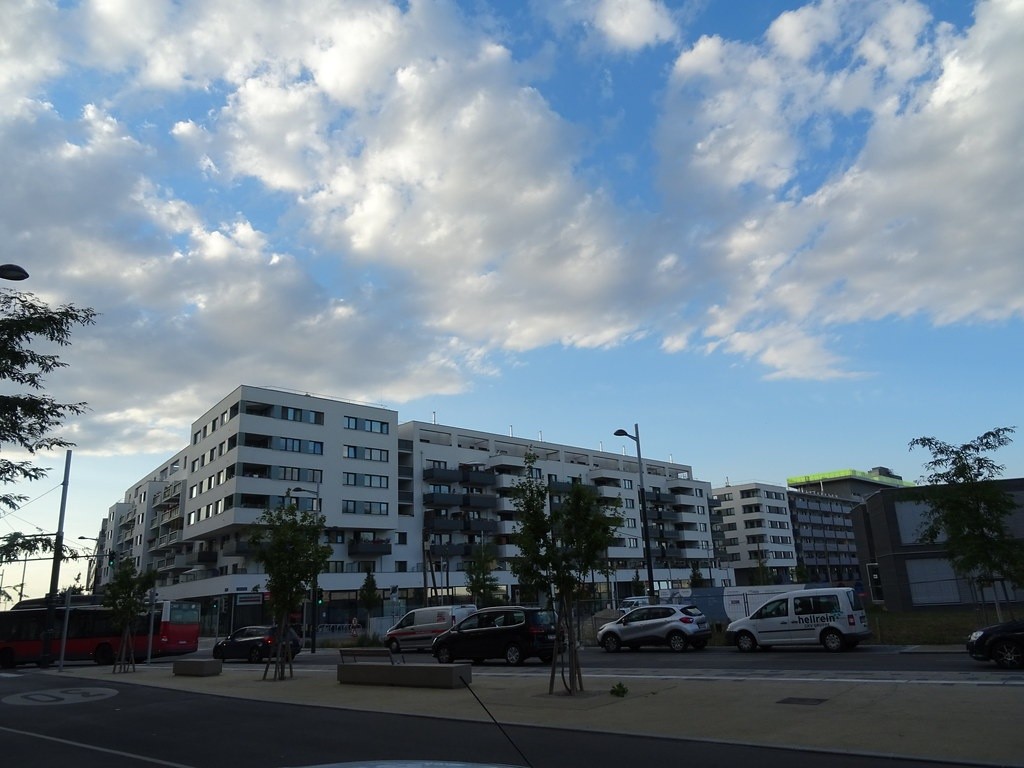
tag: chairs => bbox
[478,617,485,628]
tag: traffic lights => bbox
[109,551,115,566]
[317,588,323,605]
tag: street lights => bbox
[78,537,101,594]
[293,488,320,654]
[614,431,655,596]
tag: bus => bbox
[0,594,201,670]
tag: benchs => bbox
[339,648,406,665]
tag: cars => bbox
[966,620,1024,670]
[431,605,568,666]
[596,605,712,653]
[212,626,302,664]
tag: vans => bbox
[384,604,478,654]
[616,596,650,614]
[724,586,873,652]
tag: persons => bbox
[351,618,358,635]
